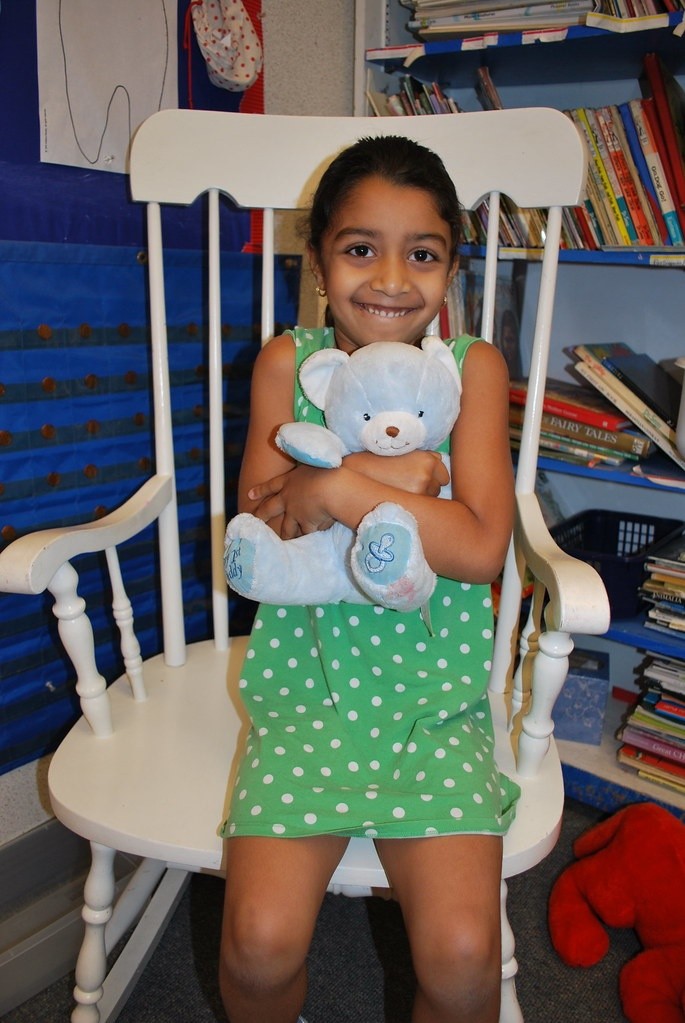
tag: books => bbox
[360,1,685,255]
[613,536,685,797]
[431,268,685,487]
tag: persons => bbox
[215,132,523,1023]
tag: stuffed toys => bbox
[222,335,462,614]
[549,802,685,1023]
